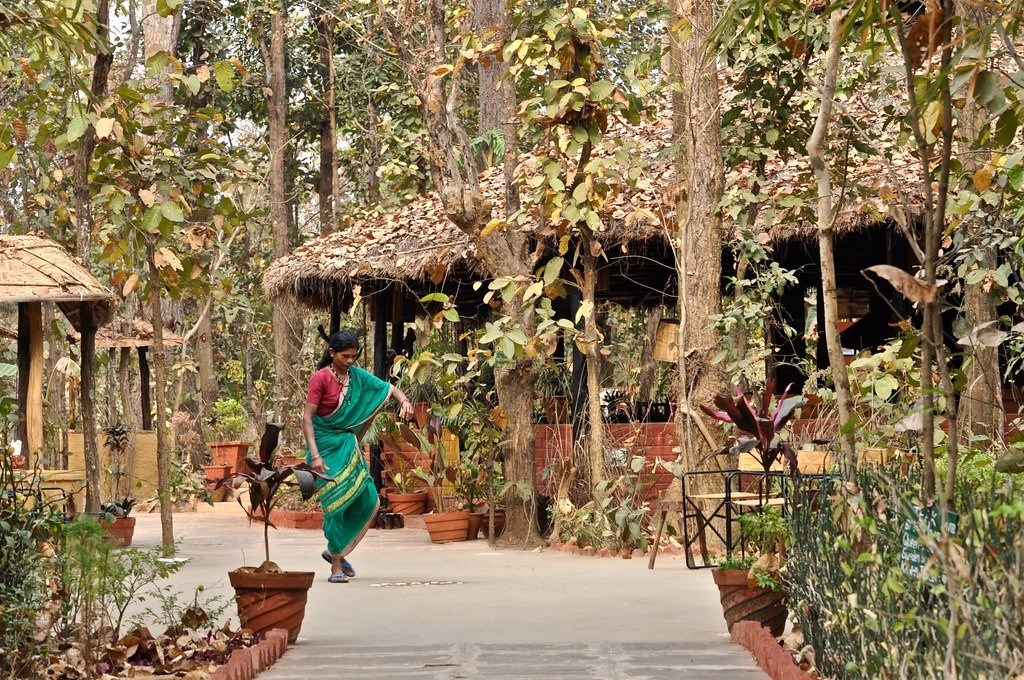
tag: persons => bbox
[302,330,415,583]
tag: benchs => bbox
[680,444,891,569]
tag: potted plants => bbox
[98,422,138,547]
[377,415,469,544]
[376,495,404,529]
[450,463,485,541]
[205,397,256,478]
[469,477,506,538]
[204,462,234,502]
[696,377,834,638]
[274,447,296,467]
[227,422,338,644]
[295,449,307,464]
[361,414,428,515]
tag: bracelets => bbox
[401,399,409,405]
[311,454,320,461]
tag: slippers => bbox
[322,551,356,577]
[328,572,350,583]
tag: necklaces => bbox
[330,364,353,405]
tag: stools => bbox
[648,500,711,570]
